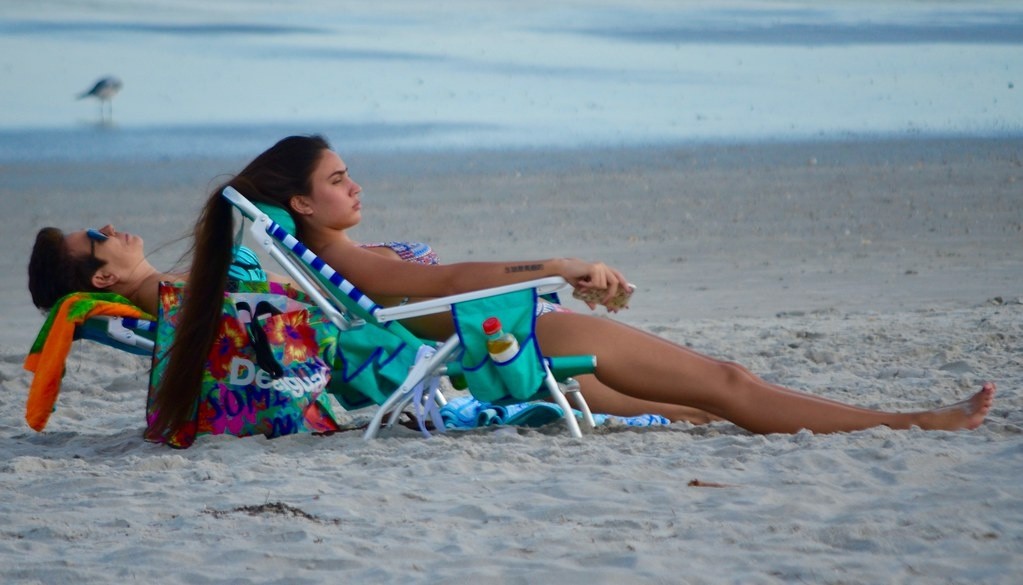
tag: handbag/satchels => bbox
[143,277,331,447]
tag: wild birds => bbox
[77,75,124,120]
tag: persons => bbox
[29,225,729,425]
[140,134,996,450]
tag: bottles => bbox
[482,318,520,364]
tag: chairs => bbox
[223,186,599,446]
[71,312,448,431]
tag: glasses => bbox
[87,228,108,274]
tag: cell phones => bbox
[572,276,637,310]
